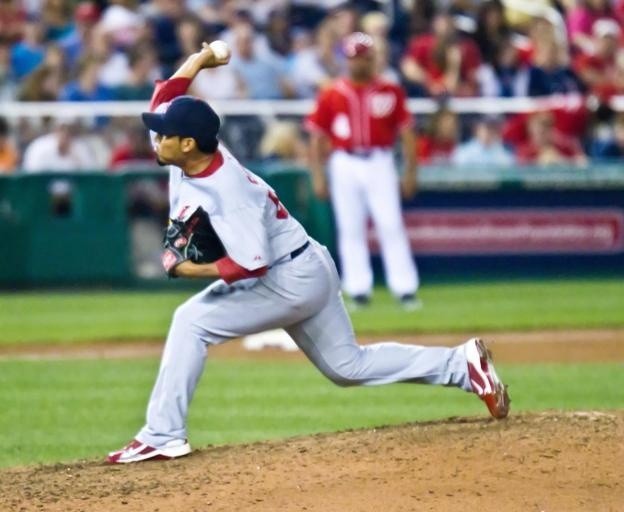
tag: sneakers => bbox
[107,438,192,464]
[346,296,372,312]
[400,293,423,314]
[464,337,510,420]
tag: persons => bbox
[98,39,510,466]
[303,31,426,313]
[1,2,623,182]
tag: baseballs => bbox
[208,40,229,59]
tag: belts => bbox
[347,147,374,159]
[290,240,311,259]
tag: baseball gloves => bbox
[163,207,227,278]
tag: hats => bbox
[343,31,376,59]
[142,95,220,139]
